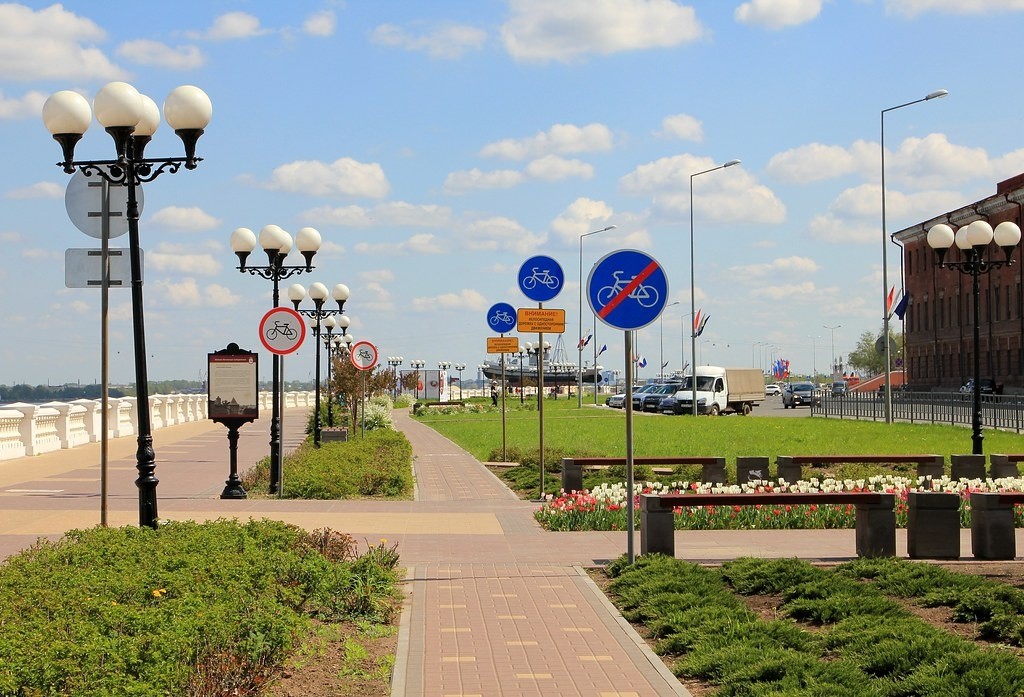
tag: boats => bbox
[480,333,603,385]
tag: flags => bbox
[583,334,593,346]
[772,359,790,380]
[894,293,909,320]
[887,285,895,313]
[640,358,647,368]
[597,344,602,357]
[578,331,589,348]
[698,316,710,337]
[694,310,700,330]
[638,356,644,368]
[599,345,607,355]
[697,314,705,332]
[890,289,903,317]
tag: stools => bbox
[906,491,962,561]
[951,454,986,483]
[736,455,770,487]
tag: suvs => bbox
[765,384,782,396]
[606,380,682,414]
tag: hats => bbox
[493,381,497,384]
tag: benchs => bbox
[425,401,465,410]
[967,493,1024,560]
[561,456,726,498]
[322,427,348,443]
[989,453,1024,480]
[776,453,947,487]
[638,492,897,560]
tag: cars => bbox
[957,377,997,403]
[781,381,822,410]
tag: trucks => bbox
[673,365,767,416]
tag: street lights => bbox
[517,341,550,410]
[822,324,842,374]
[752,341,779,377]
[437,361,466,401]
[230,224,322,493]
[660,301,680,384]
[410,359,426,400]
[926,219,1022,455]
[578,224,617,407]
[689,158,743,416]
[287,282,355,448]
[880,88,950,424]
[44,82,212,535]
[680,311,699,377]
[387,356,402,398]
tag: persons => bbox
[491,381,500,407]
[996,381,1004,402]
[505,384,508,397]
[508,385,513,397]
[515,386,520,397]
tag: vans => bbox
[831,381,850,398]
